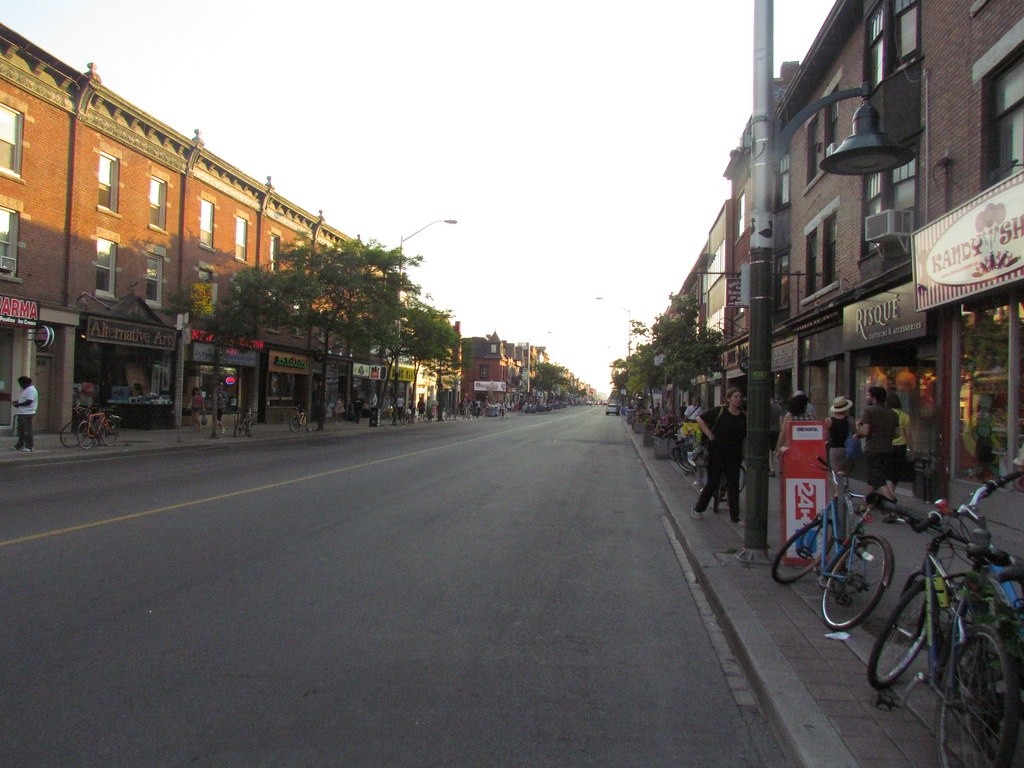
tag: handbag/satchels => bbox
[845,437,861,461]
[693,446,709,467]
[202,416,207,426]
[696,429,702,444]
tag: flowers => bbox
[631,410,682,440]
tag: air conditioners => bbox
[865,209,914,243]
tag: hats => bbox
[793,391,804,398]
[830,396,853,412]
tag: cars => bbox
[606,404,620,416]
[586,400,609,406]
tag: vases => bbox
[654,436,680,461]
[627,417,631,424]
[631,419,636,430]
[642,428,659,447]
[633,422,643,433]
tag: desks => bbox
[106,403,172,430]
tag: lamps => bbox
[345,351,352,358]
[80,332,87,338]
[323,348,332,354]
[334,351,342,356]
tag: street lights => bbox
[596,297,632,409]
[737,80,919,567]
[390,219,458,426]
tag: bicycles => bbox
[288,404,313,433]
[669,437,748,502]
[399,406,429,426]
[770,456,895,633]
[60,403,123,450]
[866,467,1024,768]
[233,409,258,438]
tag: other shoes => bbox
[23,447,33,452]
[10,447,21,451]
[882,514,896,524]
[854,508,865,515]
[729,520,745,526]
[220,427,225,435]
[691,507,704,519]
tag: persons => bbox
[852,386,914,523]
[396,394,425,421]
[650,403,660,414]
[209,388,228,434]
[324,392,345,426]
[683,396,704,423]
[824,397,860,496]
[188,387,206,430]
[679,402,687,417]
[691,388,747,527]
[9,376,38,452]
[459,395,518,420]
[776,390,817,457]
